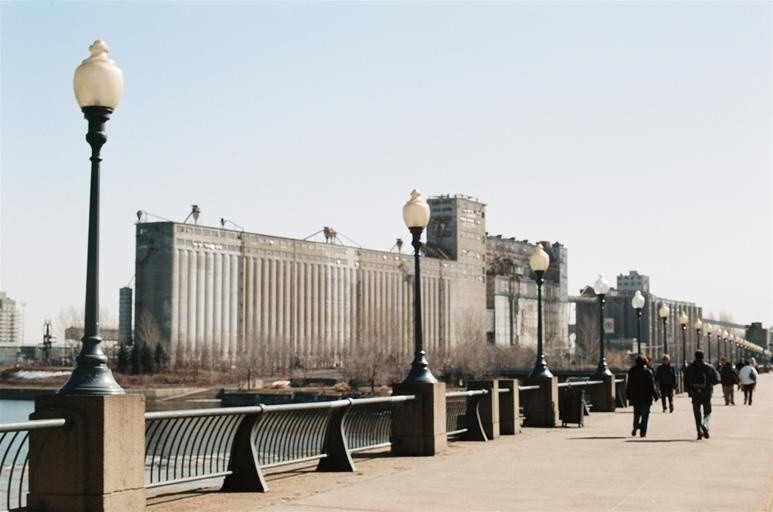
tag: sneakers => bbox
[702,423,709,439]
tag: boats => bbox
[213,366,391,417]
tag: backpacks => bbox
[691,365,708,396]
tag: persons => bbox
[679,356,759,406]
[642,355,658,389]
[655,354,676,414]
[622,354,659,436]
[683,349,718,439]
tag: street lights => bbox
[657,301,673,356]
[703,320,712,364]
[629,290,647,352]
[591,271,614,378]
[527,242,557,376]
[52,38,128,394]
[677,311,689,369]
[714,328,772,365]
[693,317,702,350]
[391,186,438,383]
